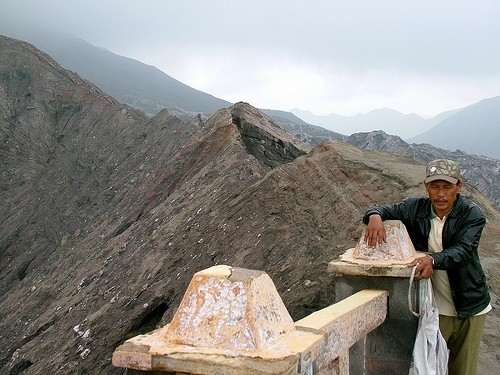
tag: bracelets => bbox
[427,254,436,271]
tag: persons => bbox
[363,159,492,375]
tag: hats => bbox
[425,159,462,184]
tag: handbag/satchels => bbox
[408,262,450,375]
